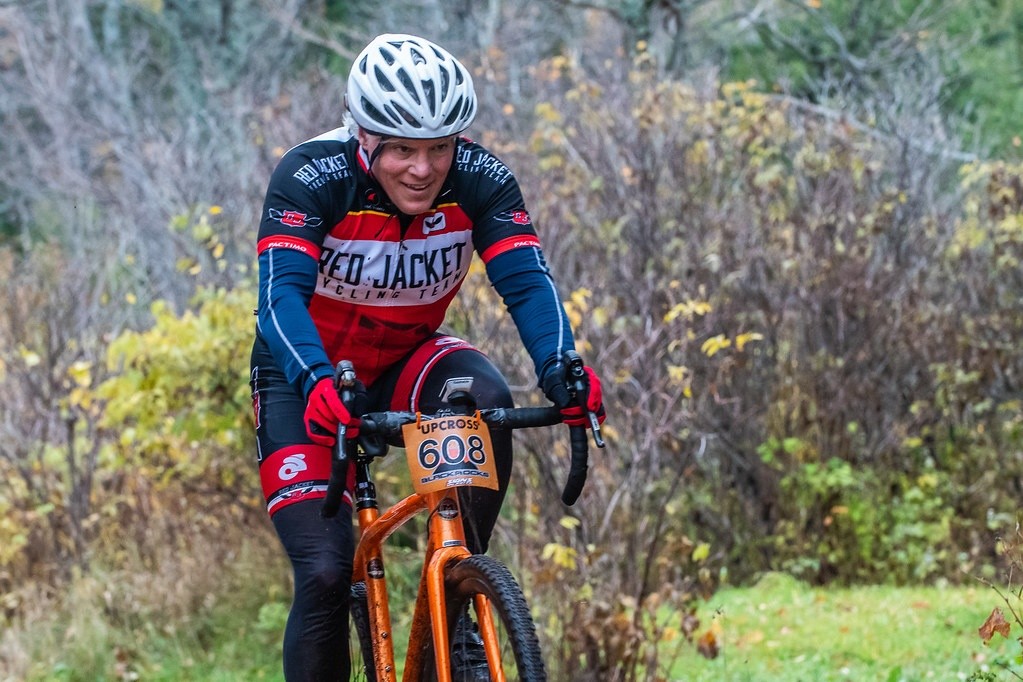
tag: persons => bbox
[250,33,605,682]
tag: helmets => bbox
[343,33,479,140]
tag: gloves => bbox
[303,375,367,446]
[543,365,606,429]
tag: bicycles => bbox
[333,351,605,682]
